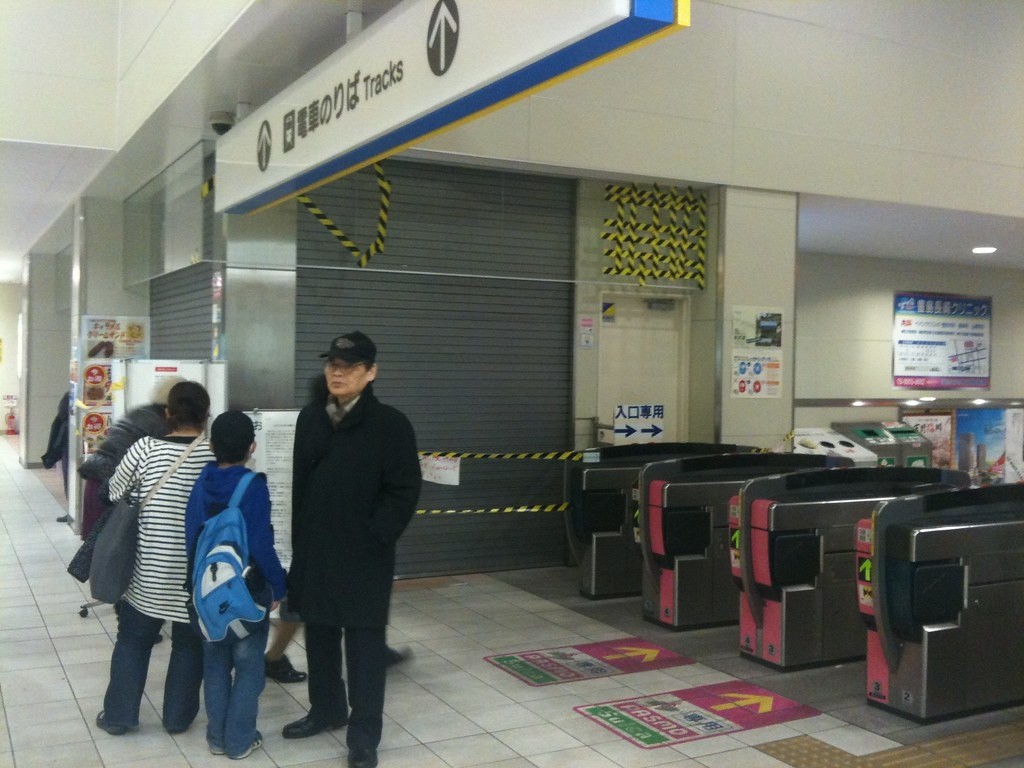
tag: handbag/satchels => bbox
[90,497,138,603]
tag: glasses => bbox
[324,359,366,371]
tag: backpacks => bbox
[183,472,273,648]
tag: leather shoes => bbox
[283,716,348,739]
[264,655,306,683]
[348,746,377,768]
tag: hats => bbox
[318,331,376,363]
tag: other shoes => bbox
[57,516,67,522]
[96,710,127,734]
[211,748,226,754]
[226,731,262,760]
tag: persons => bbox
[281,331,421,767]
[265,375,407,683]
[185,409,286,760]
[96,381,218,735]
[56,392,69,522]
[78,377,190,645]
